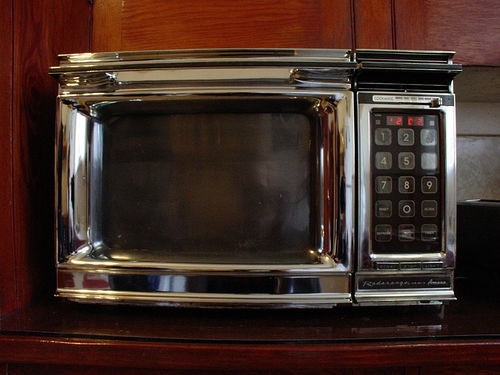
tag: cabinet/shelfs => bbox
[0,289,500,375]
[88,0,500,75]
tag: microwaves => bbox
[47,50,460,314]
